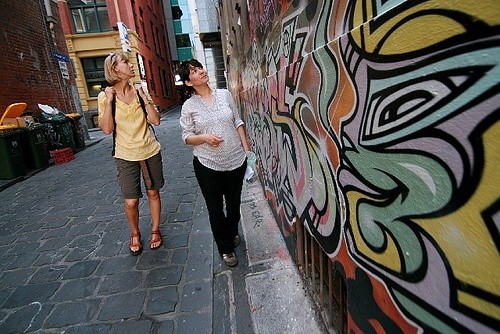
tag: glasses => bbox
[110,52,117,63]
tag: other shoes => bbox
[234,235,240,247]
[223,252,238,267]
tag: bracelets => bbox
[144,101,150,107]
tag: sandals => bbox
[150,231,162,250]
[129,231,143,256]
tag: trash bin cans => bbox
[40,113,76,153]
[65,113,86,149]
[0,102,50,181]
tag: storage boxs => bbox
[52,148,76,164]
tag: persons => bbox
[178,58,251,267]
[98,52,162,255]
